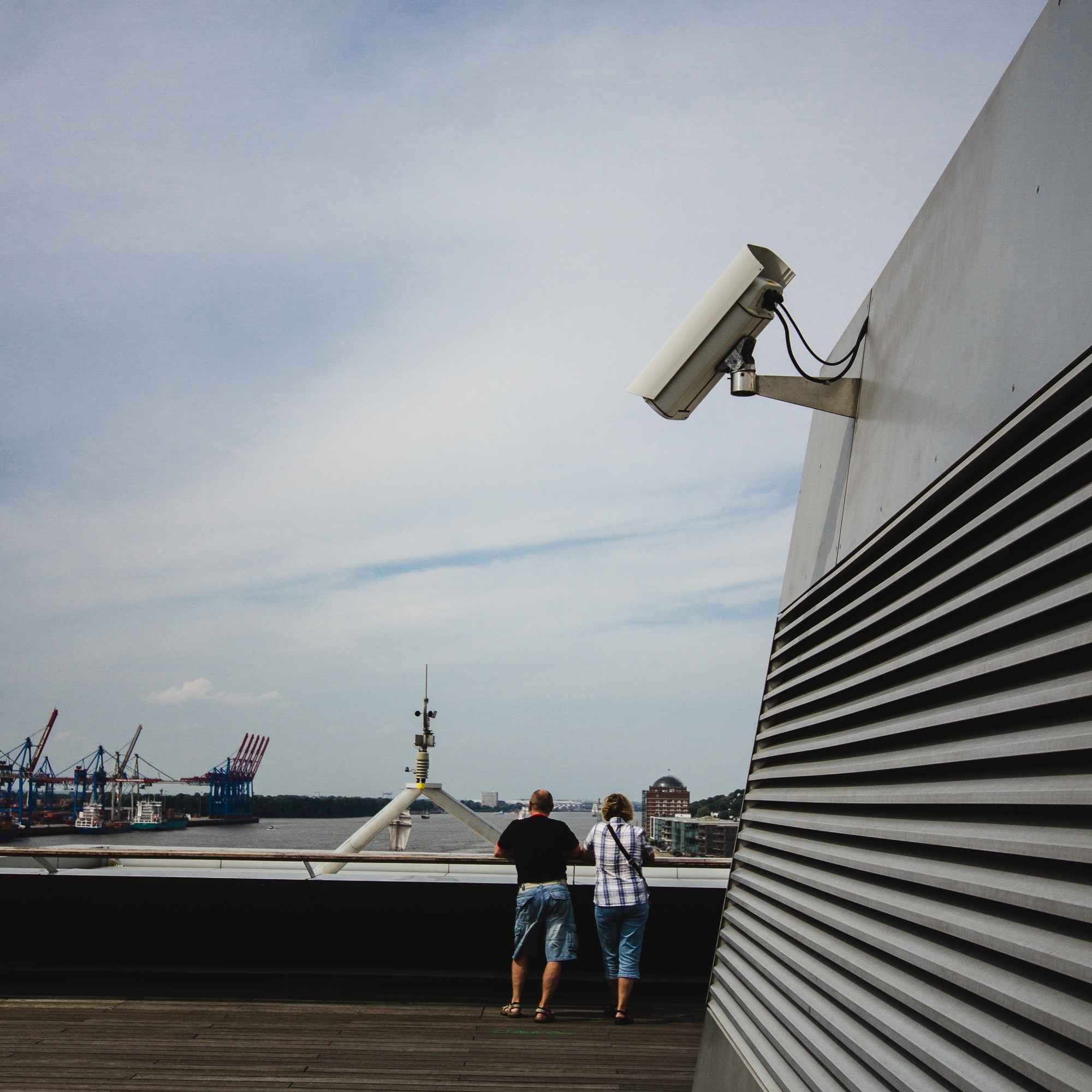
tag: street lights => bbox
[164,790,167,811]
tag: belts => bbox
[519,879,567,893]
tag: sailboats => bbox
[518,804,530,820]
[591,803,604,817]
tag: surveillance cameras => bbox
[627,244,795,420]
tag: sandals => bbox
[534,1008,555,1023]
[610,1002,617,1017]
[501,1002,522,1017]
[615,1010,634,1025]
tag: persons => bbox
[582,793,655,1024]
[493,789,581,1023]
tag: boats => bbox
[421,808,430,819]
[74,799,120,834]
[268,825,274,829]
[128,794,189,830]
[493,810,504,814]
[0,816,22,842]
